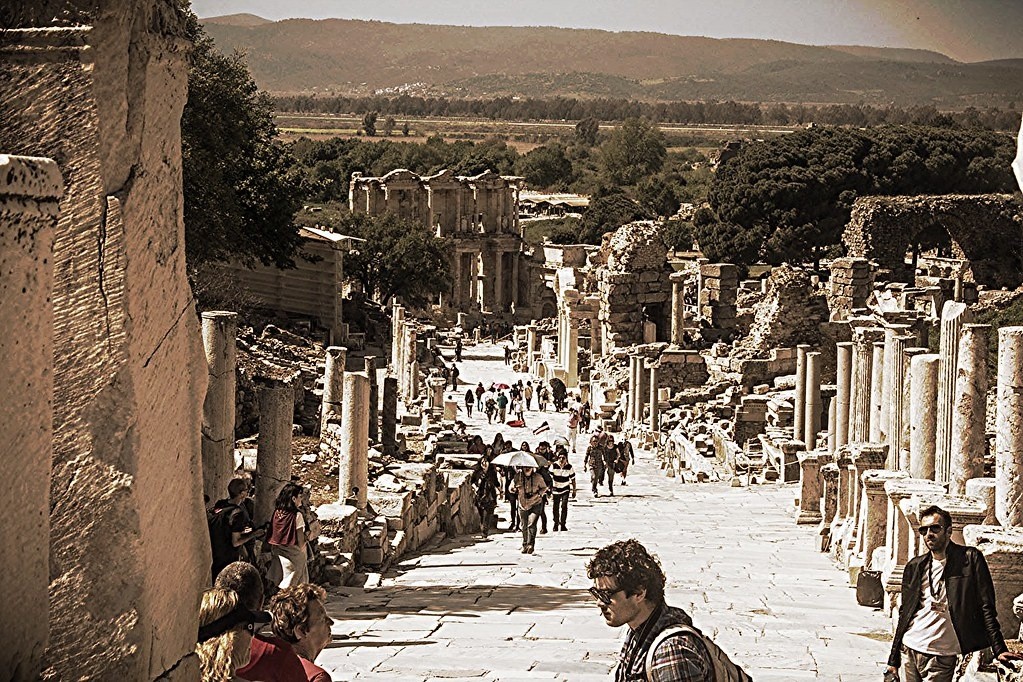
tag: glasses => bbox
[918,524,948,535]
[558,457,566,460]
[589,587,623,604]
[540,448,546,450]
[593,440,598,443]
[608,440,614,442]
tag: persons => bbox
[584,431,635,498]
[886,505,1023,682]
[198,478,334,682]
[419,364,460,392]
[454,423,577,554]
[564,397,590,454]
[446,378,567,427]
[586,539,716,682]
[454,313,516,365]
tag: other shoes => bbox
[621,480,624,485]
[561,524,568,531]
[540,529,547,534]
[527,545,533,554]
[522,542,527,553]
[599,481,603,486]
[606,493,613,497]
[594,491,598,498]
[572,449,576,453]
[482,534,488,541]
[509,523,515,530]
[516,527,521,532]
[553,525,558,531]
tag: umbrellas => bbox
[490,450,551,486]
[507,420,525,427]
[493,383,510,390]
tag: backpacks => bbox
[644,623,752,682]
[208,506,238,557]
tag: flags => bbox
[532,421,550,434]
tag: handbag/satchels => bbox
[613,460,625,473]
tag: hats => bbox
[197,602,273,643]
